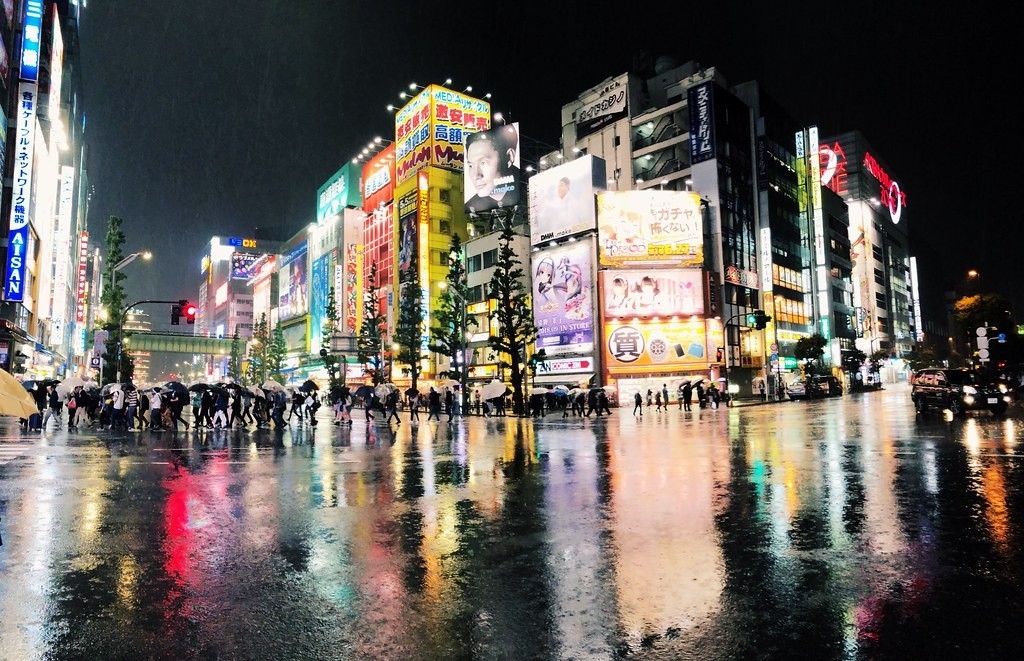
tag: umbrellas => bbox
[0,369,41,419]
[673,376,728,389]
[28,366,619,396]
[752,376,764,382]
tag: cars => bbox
[787,375,844,401]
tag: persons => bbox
[758,380,766,402]
[24,387,732,430]
[463,124,520,213]
[628,275,663,310]
[606,273,634,310]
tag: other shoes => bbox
[185,422,190,429]
[145,421,150,427]
[96,426,105,431]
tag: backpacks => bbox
[157,394,166,413]
[142,394,149,410]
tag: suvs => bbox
[912,366,1009,416]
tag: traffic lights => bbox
[748,315,761,331]
[717,351,723,362]
[186,306,196,325]
[170,304,181,326]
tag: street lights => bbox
[111,250,152,291]
[870,335,896,388]
[436,282,467,416]
[967,270,984,327]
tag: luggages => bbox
[29,400,43,431]
